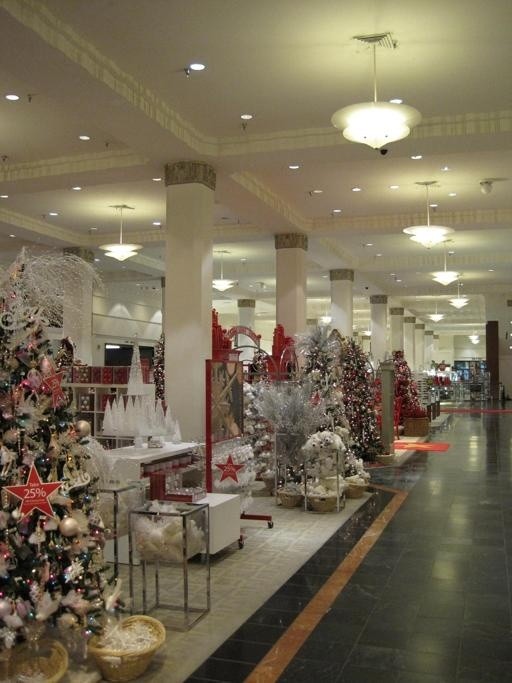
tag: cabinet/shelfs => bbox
[412,361,491,422]
[61,383,241,632]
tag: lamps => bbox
[98,205,144,261]
[331,32,422,150]
[212,251,235,291]
[402,182,492,345]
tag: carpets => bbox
[394,442,449,451]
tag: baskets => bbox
[377,453,394,466]
[263,474,275,491]
[279,487,304,507]
[1,638,69,683]
[304,491,336,513]
[86,614,167,683]
[346,478,370,498]
